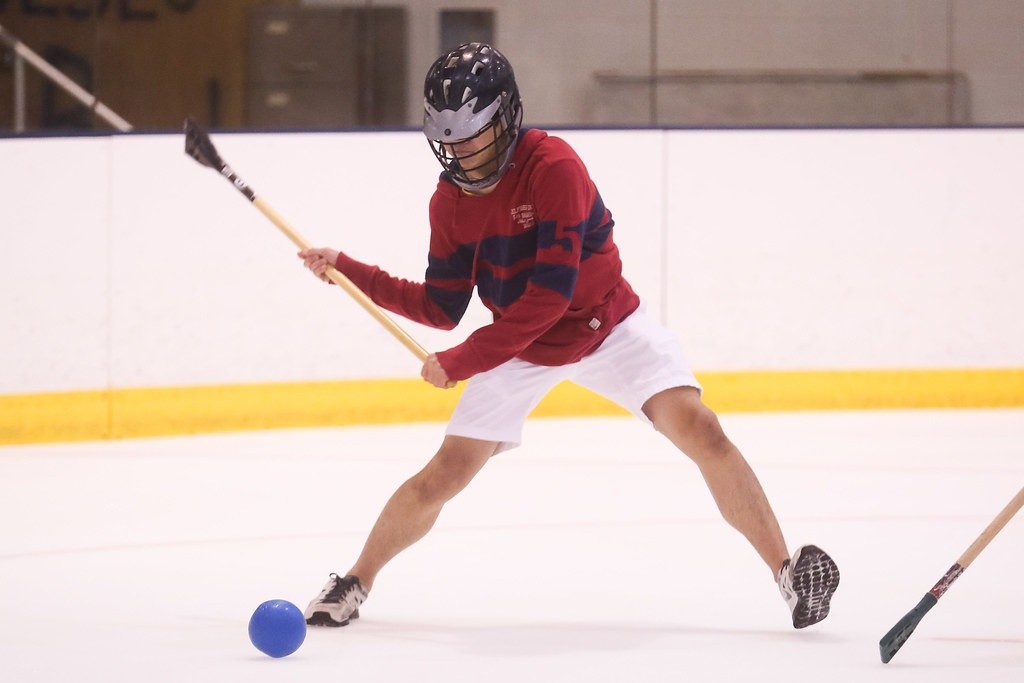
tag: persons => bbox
[297,42,838,629]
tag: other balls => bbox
[247,597,312,661]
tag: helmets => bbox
[423,42,522,190]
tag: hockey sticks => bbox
[178,110,461,391]
[875,485,1024,665]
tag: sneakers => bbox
[304,572,368,628]
[777,544,840,629]
